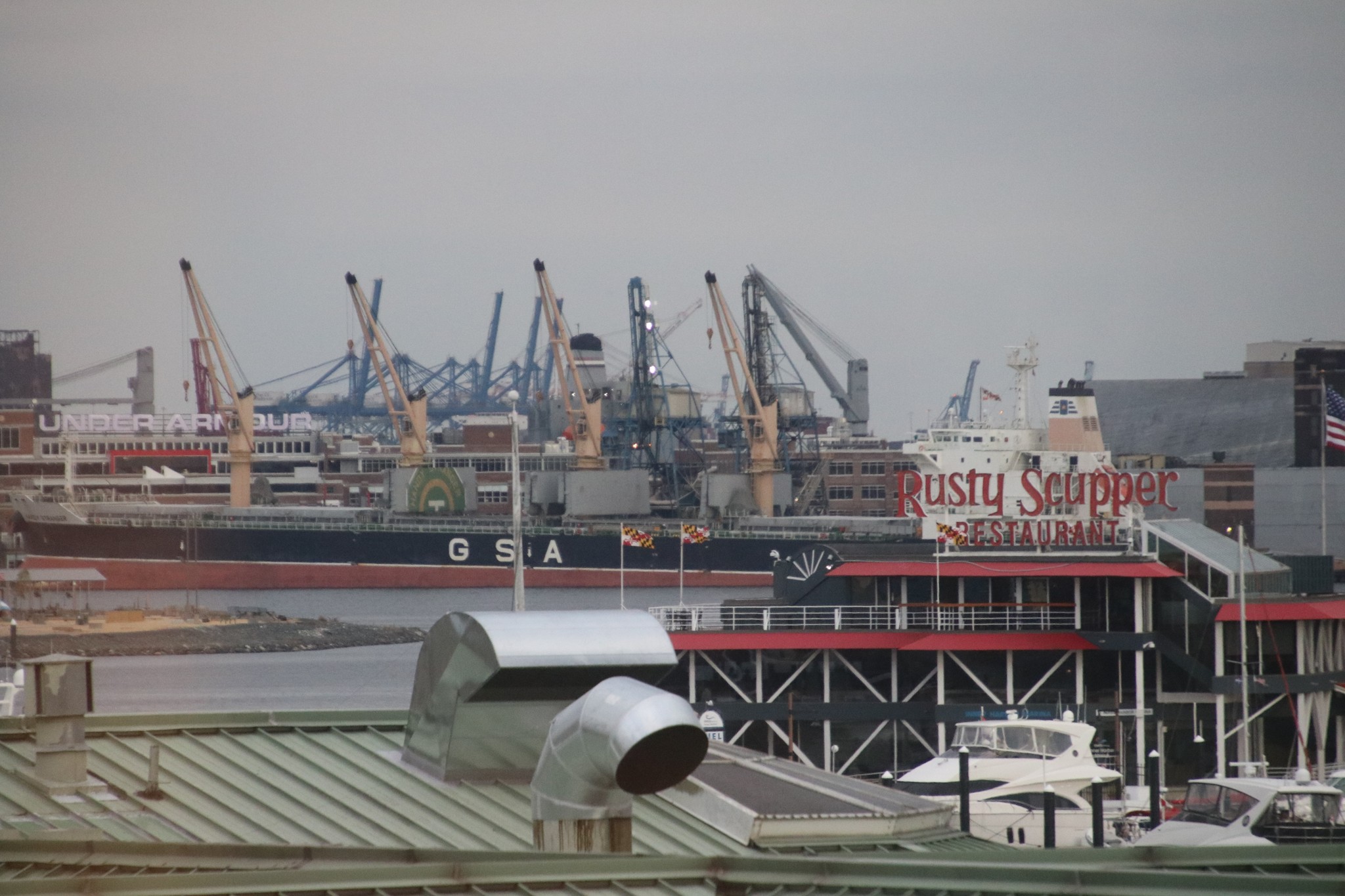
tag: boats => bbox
[896,339,1047,471]
[890,718,1344,847]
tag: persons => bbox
[1235,800,1307,827]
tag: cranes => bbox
[179,257,259,506]
[343,270,436,467]
[702,268,787,516]
[533,258,609,470]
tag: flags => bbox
[620,525,655,550]
[682,524,712,544]
[980,387,1003,402]
[1326,386,1345,449]
